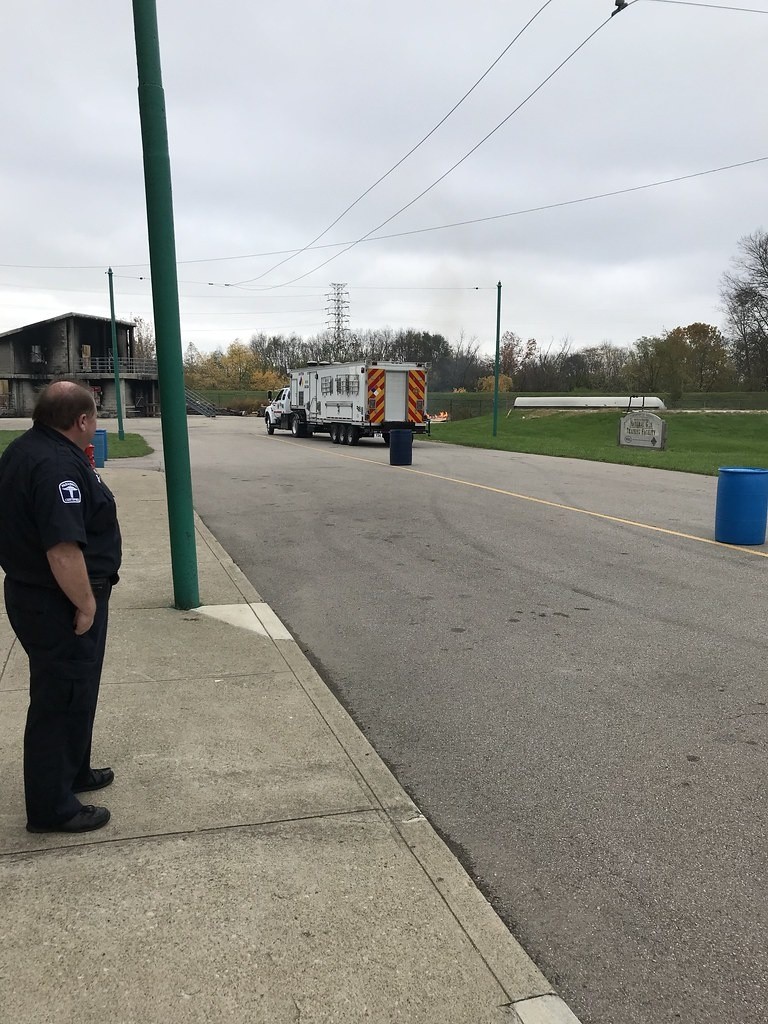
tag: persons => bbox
[0,382,122,832]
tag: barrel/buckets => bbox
[389,429,412,466]
[90,432,105,468]
[715,466,768,544]
[94,429,107,461]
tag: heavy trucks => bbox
[262,360,427,444]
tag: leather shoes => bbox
[25,804,112,835]
[71,766,115,793]
